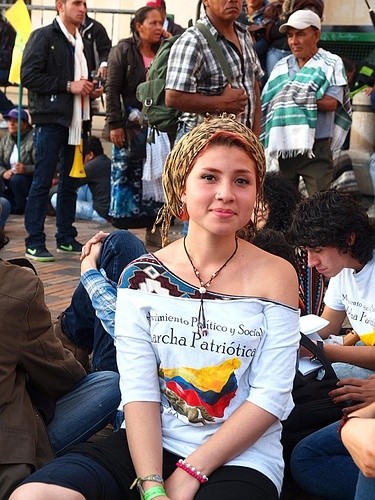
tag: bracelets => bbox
[176,462,205,483]
[130,473,164,500]
[178,459,208,481]
[67,81,71,92]
[141,486,167,500]
[339,410,360,445]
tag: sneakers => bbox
[24,246,55,261]
[57,239,84,254]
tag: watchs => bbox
[310,340,323,364]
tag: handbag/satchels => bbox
[292,331,349,429]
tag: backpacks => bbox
[136,19,235,145]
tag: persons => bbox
[165,1,265,236]
[341,57,375,193]
[20,0,104,261]
[237,0,324,93]
[105,7,173,246]
[8,112,301,500]
[0,0,185,250]
[260,10,353,198]
[0,197,121,500]
[238,173,375,500]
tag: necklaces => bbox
[184,234,238,336]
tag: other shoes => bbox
[54,315,89,368]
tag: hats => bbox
[150,111,266,250]
[2,108,29,122]
[279,9,322,34]
